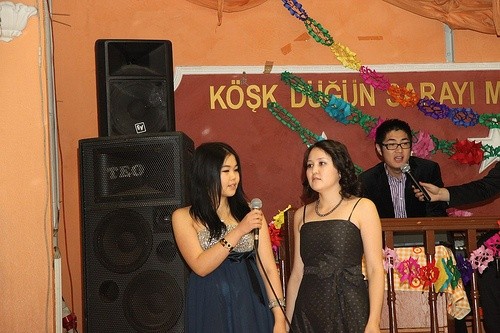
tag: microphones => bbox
[252,198,262,247]
[401,163,431,202]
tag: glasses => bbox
[381,142,412,150]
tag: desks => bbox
[377,247,461,333]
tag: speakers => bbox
[95,38,175,136]
[78,133,196,333]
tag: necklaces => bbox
[315,197,344,217]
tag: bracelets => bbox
[269,299,286,309]
[219,238,235,253]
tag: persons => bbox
[412,162,500,333]
[286,139,384,333]
[171,142,287,333]
[358,118,447,220]
